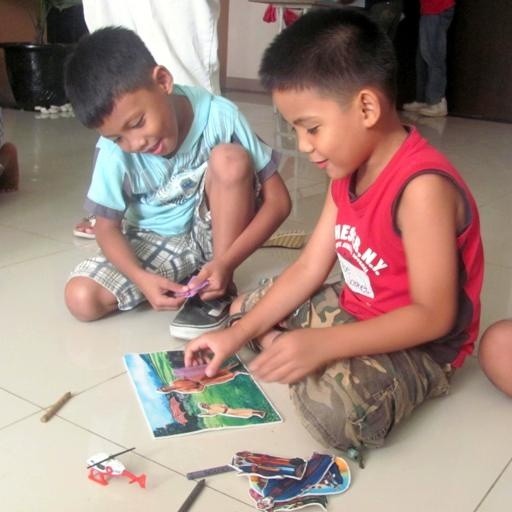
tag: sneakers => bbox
[403,96,448,117]
[169,278,238,340]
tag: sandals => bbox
[72,214,96,240]
[228,312,288,353]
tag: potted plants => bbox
[0,0,80,112]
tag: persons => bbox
[60,25,293,342]
[229,451,352,512]
[155,359,251,394]
[181,7,486,455]
[70,0,225,242]
[476,319,510,400]
[399,1,455,119]
[196,402,268,420]
